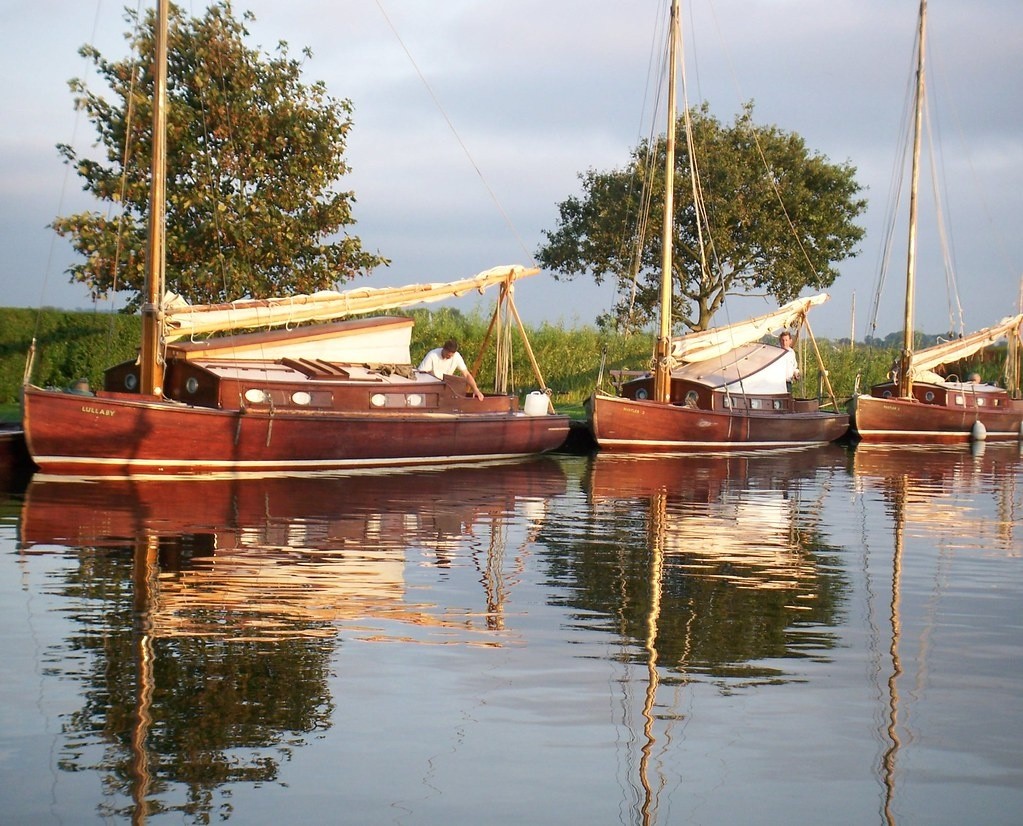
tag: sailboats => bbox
[581,445,854,826]
[15,458,570,824]
[584,0,853,454]
[21,0,574,479]
[845,1,1022,451]
[844,442,1023,825]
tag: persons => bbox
[418,341,484,402]
[968,372,981,384]
[777,332,800,410]
